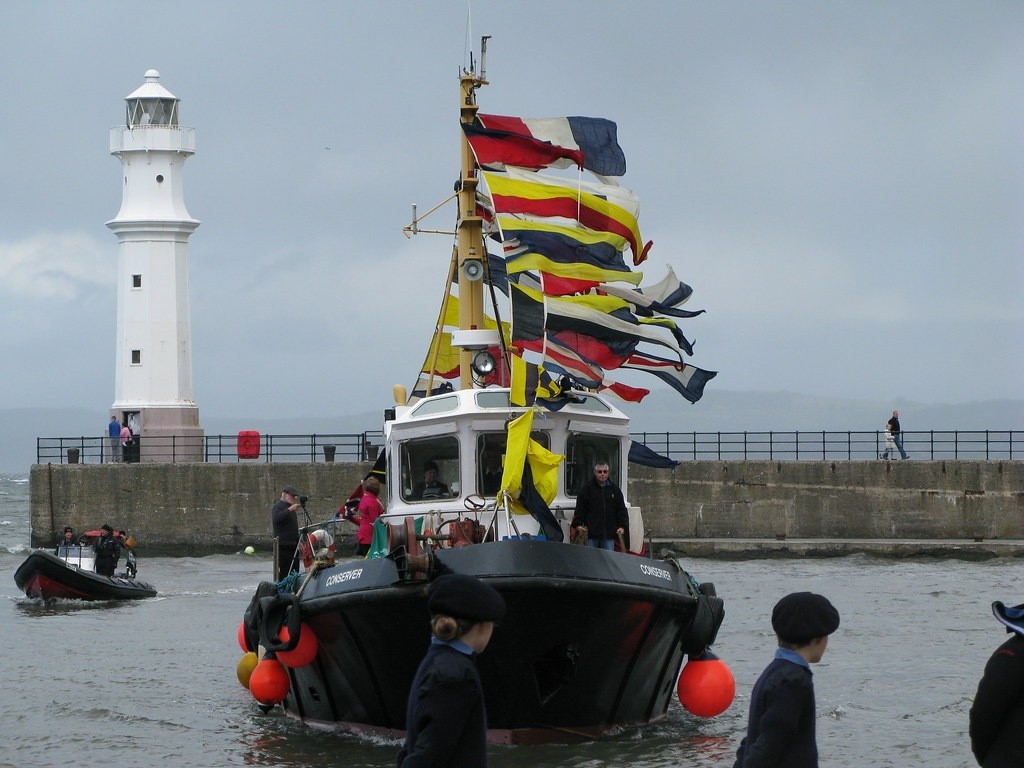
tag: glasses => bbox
[594,470,609,474]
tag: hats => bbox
[992,602,1024,637]
[771,592,839,642]
[427,573,507,623]
[423,461,438,472]
[283,487,299,498]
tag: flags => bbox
[336,113,719,519]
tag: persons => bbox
[733,592,840,768]
[354,477,386,557]
[397,574,505,768]
[409,460,451,502]
[882,411,910,460]
[572,460,628,551]
[108,415,121,465]
[478,444,504,497]
[119,422,134,464]
[271,486,303,582]
[969,601,1024,768]
[53,526,137,580]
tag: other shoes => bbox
[902,456,910,459]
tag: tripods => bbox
[287,507,315,577]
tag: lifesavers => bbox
[255,595,283,643]
[247,581,278,629]
[303,529,336,572]
[699,582,715,595]
[682,594,724,650]
[265,594,301,652]
[244,610,259,654]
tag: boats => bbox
[237,7,737,750]
[12,530,159,607]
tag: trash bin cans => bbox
[366,445,379,461]
[323,445,336,462]
[67,449,80,464]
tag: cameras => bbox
[300,496,308,507]
